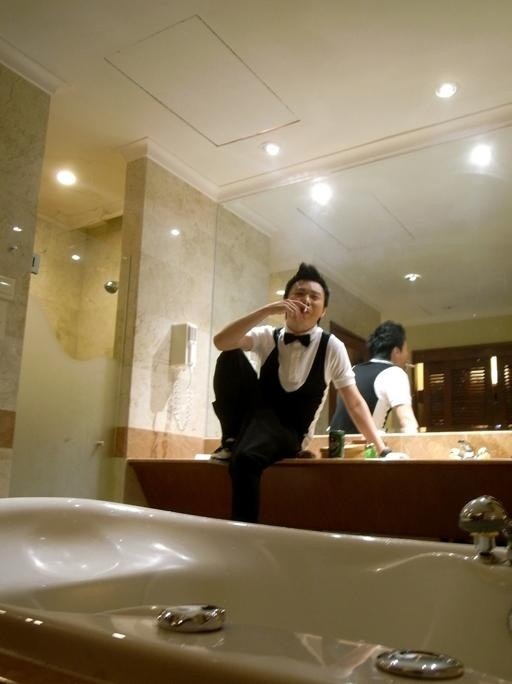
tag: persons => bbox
[213,260,412,522]
[327,318,419,435]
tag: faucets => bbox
[457,439,474,460]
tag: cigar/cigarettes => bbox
[303,308,307,313]
[406,363,416,368]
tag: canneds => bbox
[329,429,345,456]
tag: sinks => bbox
[0,497,510,682]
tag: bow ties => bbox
[284,333,310,347]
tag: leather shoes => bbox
[210,441,238,464]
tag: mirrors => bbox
[204,118,511,439]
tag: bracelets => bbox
[380,449,392,457]
